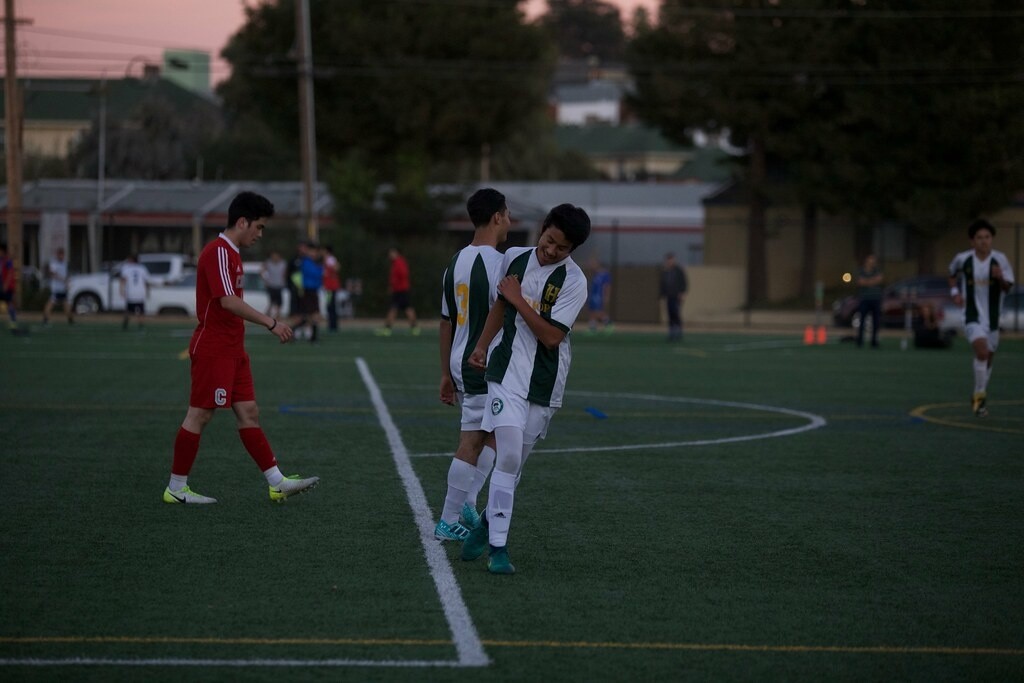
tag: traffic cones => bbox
[817,326,828,345]
[803,325,815,345]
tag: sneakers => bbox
[459,501,480,531]
[486,541,516,574]
[268,475,320,503]
[163,485,217,504]
[460,509,490,561]
[435,519,471,540]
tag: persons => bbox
[459,204,587,570]
[586,255,615,342]
[376,247,421,339]
[949,220,1015,415]
[1,235,23,331]
[159,191,318,504]
[433,184,512,539]
[261,239,341,343]
[118,254,147,330]
[853,254,885,351]
[657,250,690,339]
[42,246,75,327]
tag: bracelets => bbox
[268,319,277,331]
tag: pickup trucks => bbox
[64,252,198,317]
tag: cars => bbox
[830,273,953,330]
[143,259,352,320]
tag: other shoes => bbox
[410,326,420,336]
[971,392,989,418]
[374,328,392,337]
